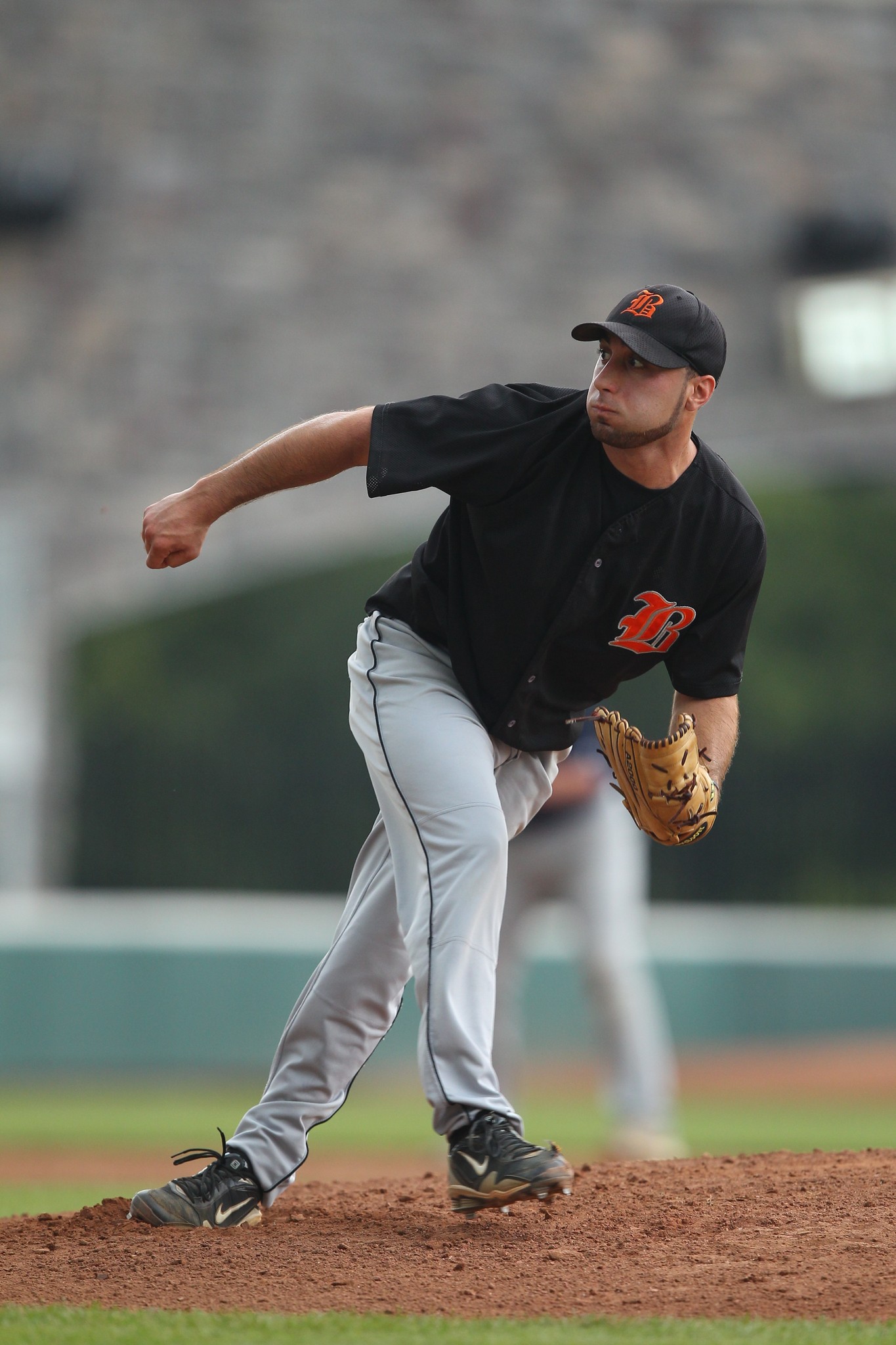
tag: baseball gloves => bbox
[561,703,722,849]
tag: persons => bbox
[510,706,684,1156]
[131,283,767,1227]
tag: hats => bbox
[571,284,727,389]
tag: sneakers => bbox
[126,1126,263,1231]
[447,1108,575,1220]
